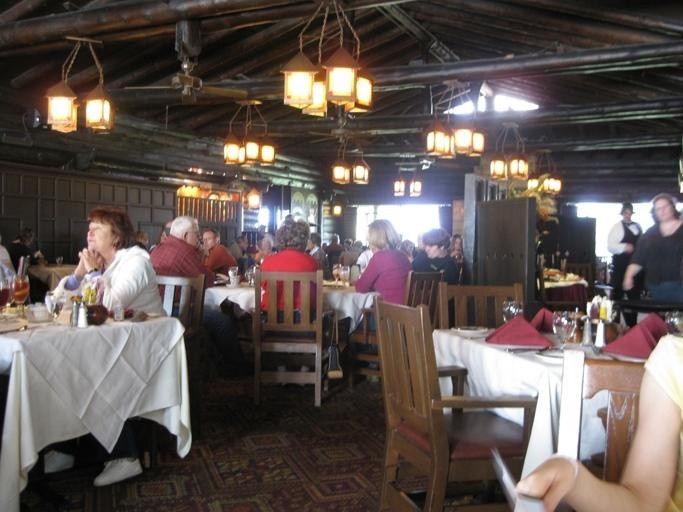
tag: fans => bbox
[112,18,249,102]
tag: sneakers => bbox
[92,458,143,486]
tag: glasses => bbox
[189,230,202,235]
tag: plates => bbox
[605,351,650,363]
[486,339,552,352]
[0,317,28,332]
[453,324,490,339]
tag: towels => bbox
[528,307,560,333]
[638,313,668,341]
[486,315,551,346]
[602,324,656,358]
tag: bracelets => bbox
[546,453,579,477]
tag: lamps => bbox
[246,183,260,209]
[48,36,115,133]
[392,157,421,197]
[331,197,340,215]
[489,121,528,181]
[527,149,560,193]
[425,78,485,160]
[279,0,373,117]
[223,99,275,167]
[331,137,370,185]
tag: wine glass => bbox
[340,265,350,288]
[45,292,67,326]
[332,264,340,287]
[245,268,255,287]
[664,312,683,337]
[10,274,30,328]
[228,266,238,286]
[0,279,15,322]
[502,300,524,334]
[56,256,63,267]
[553,312,576,356]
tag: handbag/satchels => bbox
[328,313,345,379]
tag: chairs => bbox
[435,282,523,329]
[369,297,536,512]
[348,270,441,389]
[154,275,205,320]
[247,270,323,406]
[568,259,591,301]
[560,352,660,488]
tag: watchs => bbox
[88,268,100,275]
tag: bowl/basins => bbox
[229,275,241,285]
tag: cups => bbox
[114,308,124,320]
[25,302,49,322]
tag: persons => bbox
[449,234,465,275]
[356,219,414,383]
[203,228,246,284]
[46,206,169,485]
[307,233,330,279]
[240,245,256,273]
[0,244,19,302]
[6,227,44,300]
[247,218,329,386]
[322,234,371,267]
[228,236,250,259]
[149,216,253,379]
[414,229,460,285]
[623,193,683,303]
[251,235,279,265]
[536,219,568,270]
[160,222,171,244]
[607,203,644,311]
[127,231,150,252]
[517,332,683,512]
[401,239,418,263]
